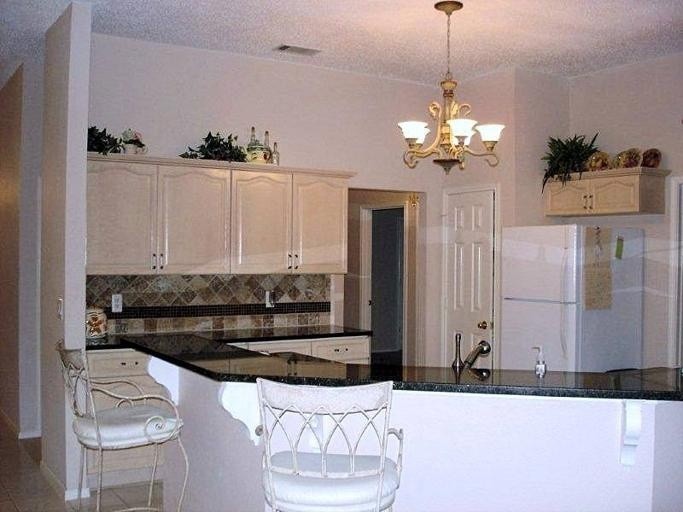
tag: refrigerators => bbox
[499,223,644,373]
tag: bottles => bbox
[84,308,108,340]
[270,142,278,165]
[249,126,256,144]
[264,131,272,163]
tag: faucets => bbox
[450,333,492,368]
[453,368,491,385]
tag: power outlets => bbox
[110,294,122,313]
[264,290,275,308]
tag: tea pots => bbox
[121,129,147,154]
[239,144,270,164]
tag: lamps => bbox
[395,1,507,175]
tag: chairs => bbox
[48,344,190,512]
[253,376,405,511]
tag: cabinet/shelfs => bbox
[83,151,233,276]
[231,162,358,273]
[310,334,370,363]
[87,350,172,489]
[250,336,312,356]
[543,168,672,219]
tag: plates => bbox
[587,149,662,172]
